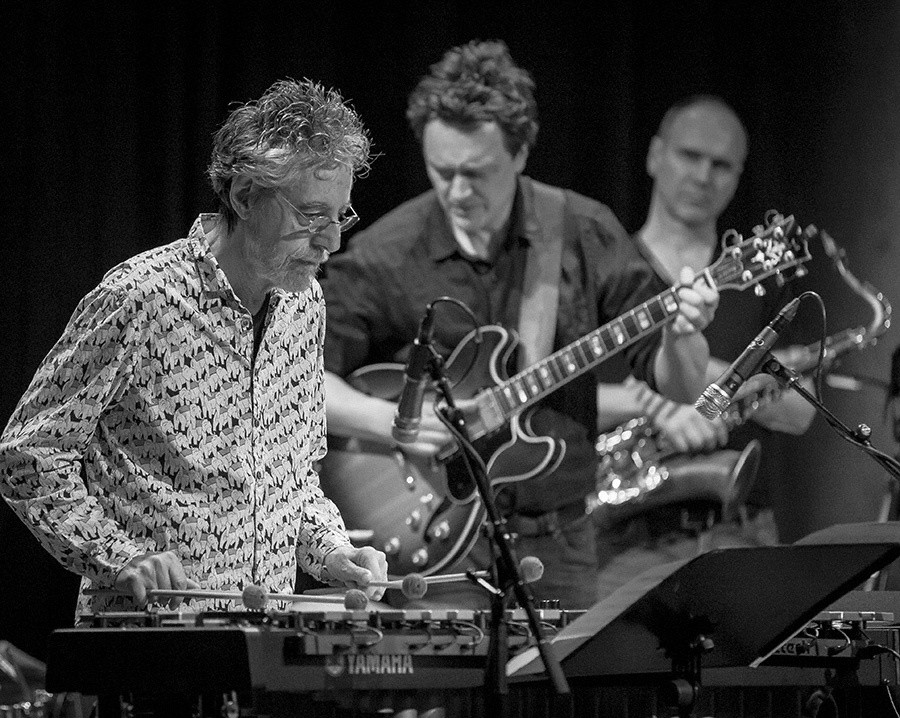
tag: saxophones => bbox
[587,231,894,532]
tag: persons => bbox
[1,76,388,630]
[597,94,818,603]
[319,39,720,627]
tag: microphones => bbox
[391,304,435,444]
[693,297,801,421]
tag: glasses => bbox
[270,186,360,235]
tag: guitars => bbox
[316,212,816,579]
